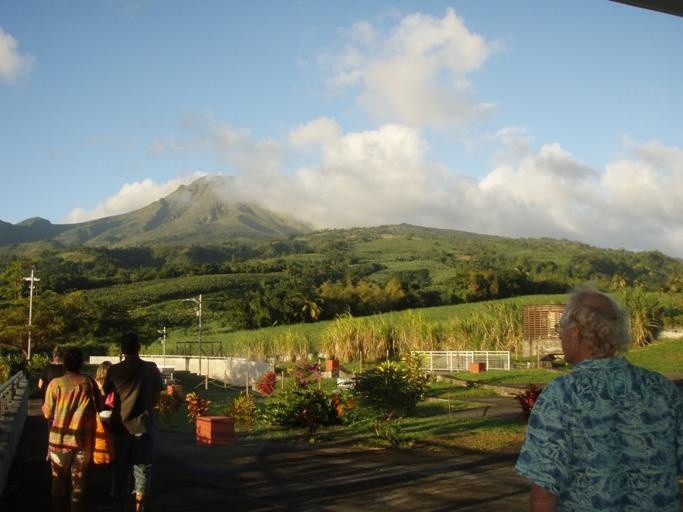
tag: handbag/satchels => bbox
[87,377,117,466]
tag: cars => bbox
[540,352,567,368]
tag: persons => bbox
[37,344,65,402]
[98,328,162,511]
[93,359,113,431]
[37,345,105,510]
[510,291,682,511]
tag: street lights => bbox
[22,275,41,362]
[156,325,165,369]
[182,298,201,376]
